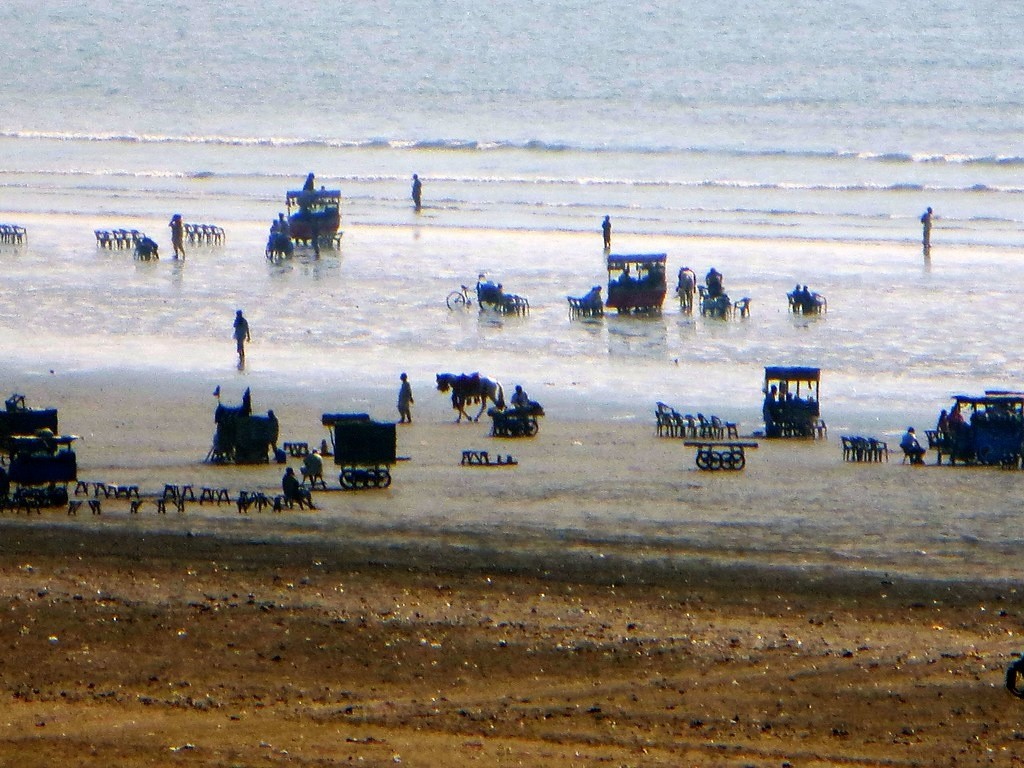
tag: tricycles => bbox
[265,236,294,263]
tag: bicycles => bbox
[446,282,497,311]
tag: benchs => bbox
[459,449,491,466]
[283,442,309,456]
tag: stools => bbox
[10,480,312,515]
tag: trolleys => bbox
[133,231,160,260]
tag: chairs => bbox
[813,418,827,439]
[787,292,828,316]
[0,225,27,244]
[502,294,530,317]
[697,285,732,322]
[94,229,159,261]
[567,296,603,323]
[842,436,888,462]
[182,224,226,245]
[899,442,914,465]
[655,401,740,440]
[734,297,750,319]
[334,232,343,243]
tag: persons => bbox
[902,427,922,464]
[706,268,722,296]
[282,467,317,510]
[233,310,250,358]
[266,409,279,460]
[309,218,321,256]
[168,214,186,260]
[792,284,810,302]
[310,449,323,482]
[590,288,605,315]
[493,386,533,436]
[477,275,503,312]
[270,213,290,235]
[398,373,413,423]
[602,216,611,250]
[412,174,422,211]
[22,427,58,457]
[938,406,964,439]
[619,263,659,285]
[303,173,315,190]
[923,207,934,248]
[763,385,792,418]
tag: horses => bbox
[675,267,697,311]
[436,373,508,423]
